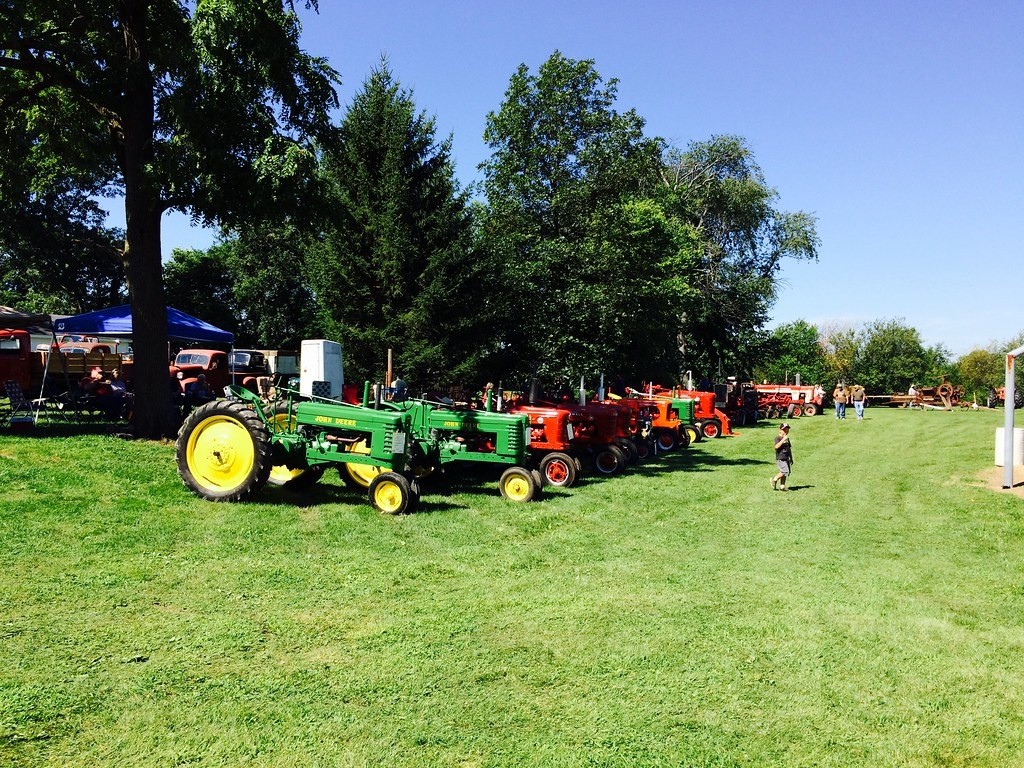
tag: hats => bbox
[839,384,842,388]
[91,366,104,374]
[911,384,915,388]
[853,385,859,387]
[779,423,790,430]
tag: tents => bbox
[0,306,51,393]
[36,304,234,421]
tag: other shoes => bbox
[779,487,789,491]
[770,478,777,490]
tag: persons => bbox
[909,385,919,407]
[972,402,978,410]
[391,373,408,399]
[82,367,134,420]
[770,422,793,491]
[482,382,496,409]
[814,384,826,415]
[191,374,216,400]
[850,385,868,420]
[833,383,848,420]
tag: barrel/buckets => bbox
[995,427,1024,467]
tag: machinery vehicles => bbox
[0,318,826,516]
[987,384,1022,410]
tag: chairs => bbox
[28,362,71,423]
[2,380,50,427]
[182,382,215,415]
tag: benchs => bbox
[76,376,133,420]
